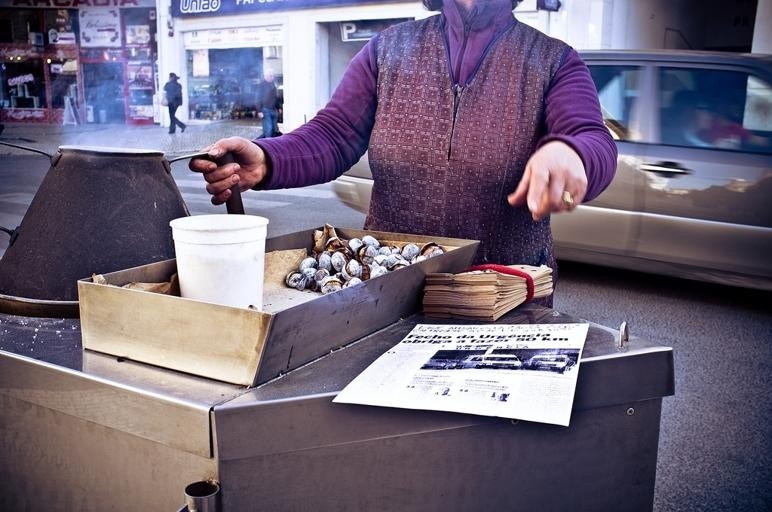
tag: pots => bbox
[0,140,208,314]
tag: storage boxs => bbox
[77,226,481,388]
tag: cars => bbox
[332,50,771,296]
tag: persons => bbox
[670,82,771,154]
[189,1,619,308]
[164,73,186,134]
[255,68,280,137]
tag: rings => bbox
[561,187,577,207]
